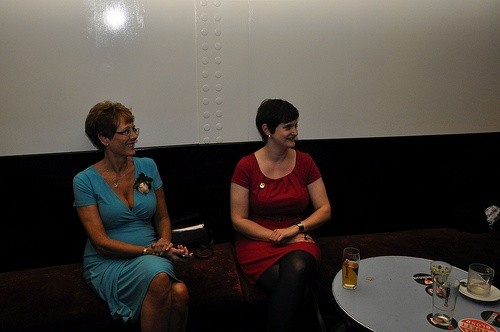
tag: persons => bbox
[230,99,330,332]
[72,101,193,332]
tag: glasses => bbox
[114,127,139,137]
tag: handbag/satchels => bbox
[171,223,215,259]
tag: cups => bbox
[430,261,451,293]
[342,247,360,290]
[432,275,461,326]
[467,263,494,297]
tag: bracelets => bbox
[295,221,304,232]
[143,246,148,255]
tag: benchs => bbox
[0,132,500,332]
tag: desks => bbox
[332,255,500,332]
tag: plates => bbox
[455,279,500,302]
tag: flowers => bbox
[132,172,154,194]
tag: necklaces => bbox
[103,160,131,187]
